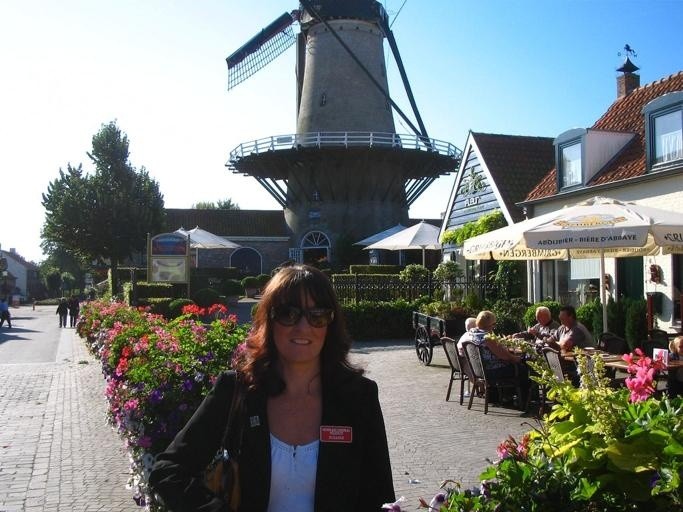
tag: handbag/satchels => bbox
[197,451,241,511]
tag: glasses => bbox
[269,305,335,328]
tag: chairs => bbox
[438,329,671,421]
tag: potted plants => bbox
[241,274,271,298]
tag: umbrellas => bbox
[362,218,441,270]
[182,225,242,269]
[173,225,188,235]
[462,192,683,340]
[352,223,408,266]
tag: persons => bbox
[54,297,70,328]
[67,295,79,328]
[149,264,396,510]
[506,305,559,356]
[666,335,682,398]
[0,298,12,329]
[455,317,480,375]
[548,306,596,389]
[468,310,526,411]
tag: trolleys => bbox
[410,310,469,369]
